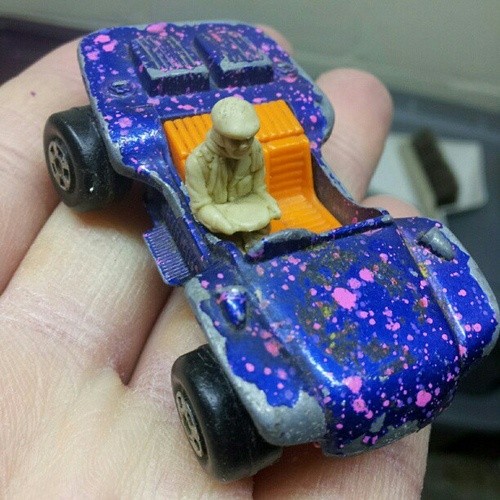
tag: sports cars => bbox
[42,19,500,483]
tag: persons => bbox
[0,18,435,500]
[184,97,281,253]
